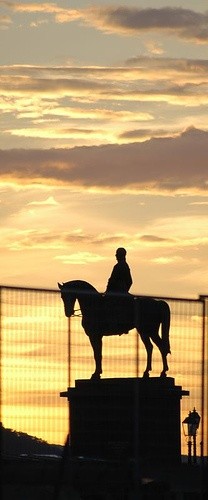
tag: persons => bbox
[104,247,133,335]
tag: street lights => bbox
[182,406,201,465]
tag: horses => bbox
[58,280,171,379]
